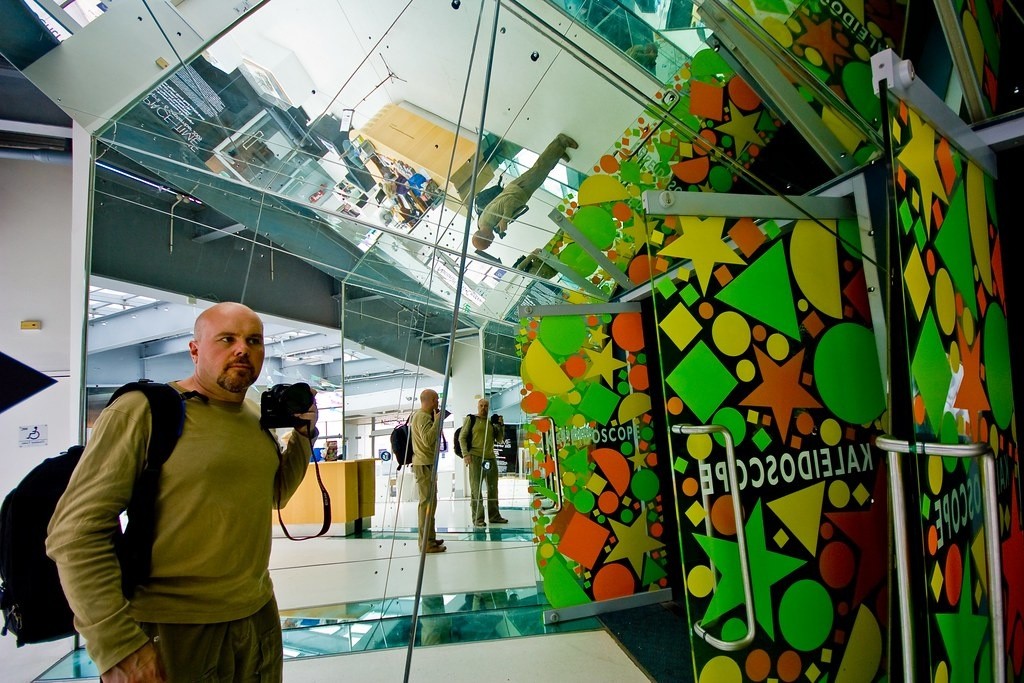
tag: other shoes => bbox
[434,538,445,547]
[556,133,578,150]
[561,151,571,163]
[490,516,509,523]
[421,545,446,554]
[473,516,487,527]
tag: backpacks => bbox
[390,413,413,471]
[474,176,506,231]
[0,377,207,646]
[454,414,475,458]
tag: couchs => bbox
[257,92,378,194]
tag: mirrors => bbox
[87,1,692,627]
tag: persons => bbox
[472,132,579,251]
[46,302,320,683]
[457,398,509,527]
[517,248,557,279]
[408,388,447,552]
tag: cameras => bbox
[494,226,506,239]
[260,382,314,429]
[491,414,501,425]
[431,410,439,422]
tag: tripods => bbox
[340,53,407,132]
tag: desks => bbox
[272,460,363,537]
[354,100,499,221]
[351,458,382,533]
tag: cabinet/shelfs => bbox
[236,34,317,113]
